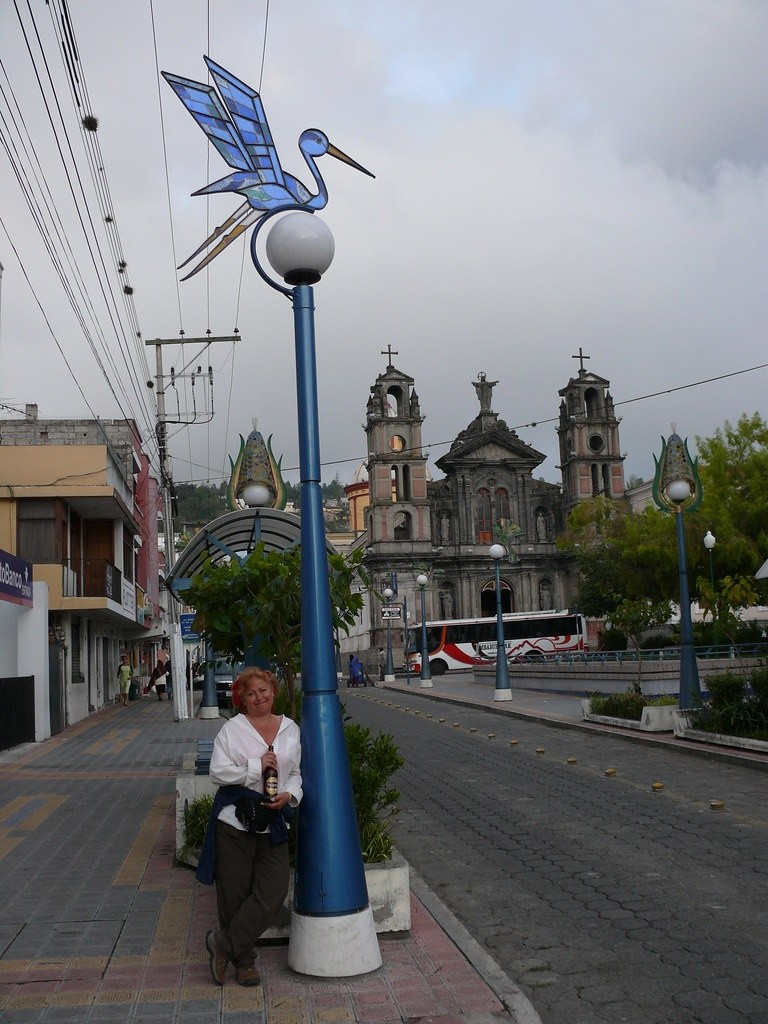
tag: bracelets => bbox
[288,792,292,801]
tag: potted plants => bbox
[639,693,681,731]
[255,683,412,937]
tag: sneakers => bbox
[236,959,260,986]
[206,930,230,986]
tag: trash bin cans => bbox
[128,679,141,700]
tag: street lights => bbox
[383,589,397,681]
[227,416,288,510]
[488,544,513,703]
[164,53,385,977]
[416,575,433,688]
[703,530,716,621]
[651,423,703,710]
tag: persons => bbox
[379,647,386,681]
[153,655,173,701]
[186,661,195,690]
[205,667,303,986]
[117,655,133,706]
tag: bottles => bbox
[263,745,278,804]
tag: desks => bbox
[133,676,157,696]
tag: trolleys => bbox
[347,660,367,687]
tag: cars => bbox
[214,658,245,709]
[194,671,205,690]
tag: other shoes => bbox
[125,702,128,706]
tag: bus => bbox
[400,608,588,675]
